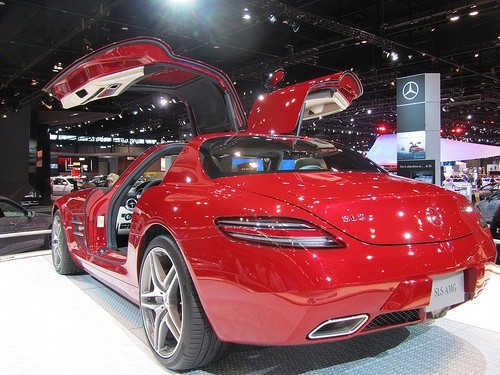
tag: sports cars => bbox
[37,35,462,373]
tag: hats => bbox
[106,173,119,182]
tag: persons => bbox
[73,178,78,191]
[50,179,54,197]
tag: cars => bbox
[442,170,500,240]
[0,192,52,257]
[48,171,116,198]
